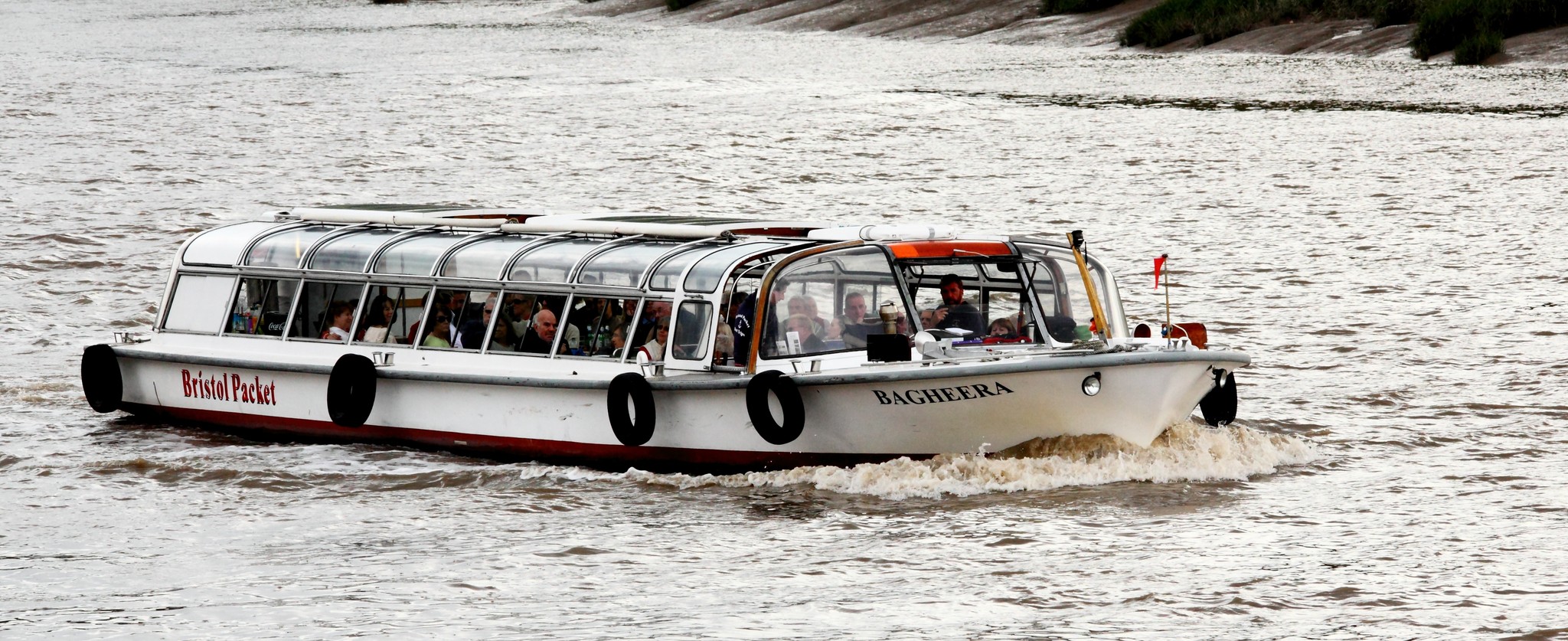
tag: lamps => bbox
[1081,372,1101,396]
[1206,365,1227,388]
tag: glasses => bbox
[786,326,805,332]
[436,316,452,323]
[484,310,493,314]
[656,325,668,331]
[511,299,529,305]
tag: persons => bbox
[322,270,1016,364]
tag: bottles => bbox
[584,325,609,352]
[236,301,264,335]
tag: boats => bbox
[79,200,1254,476]
